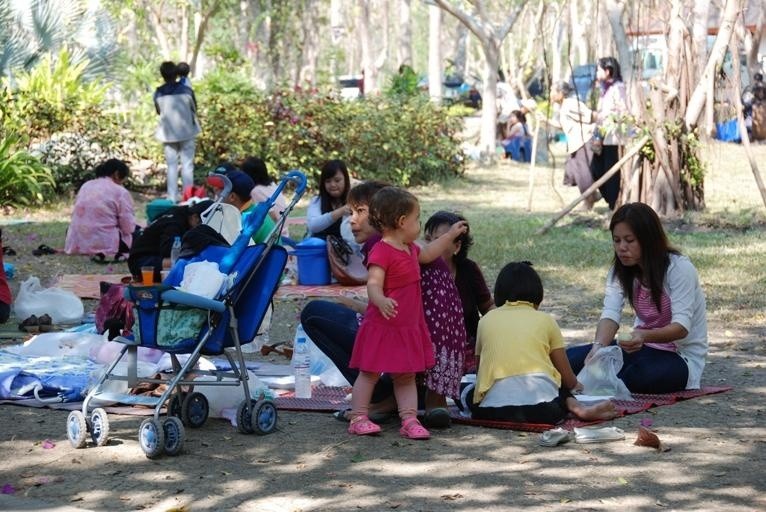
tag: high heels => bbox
[284,348,294,357]
[262,341,293,355]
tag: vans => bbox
[326,74,366,104]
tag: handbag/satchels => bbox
[328,235,368,285]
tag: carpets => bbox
[263,356,734,433]
[58,263,370,303]
[0,320,300,417]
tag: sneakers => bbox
[39,313,51,331]
[19,315,40,333]
[574,427,624,443]
[539,428,569,447]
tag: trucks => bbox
[626,36,749,87]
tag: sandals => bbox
[348,416,381,435]
[400,418,430,438]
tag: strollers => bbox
[144,185,210,224]
[67,169,307,453]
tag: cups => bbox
[160,271,170,282]
[141,266,154,287]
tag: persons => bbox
[64,158,145,256]
[551,58,633,209]
[452,261,619,425]
[184,157,289,245]
[740,71,766,143]
[301,159,496,440]
[566,202,710,393]
[495,67,543,162]
[153,61,202,201]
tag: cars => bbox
[568,64,596,100]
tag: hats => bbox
[208,171,254,195]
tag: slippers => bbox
[32,244,58,255]
[333,409,376,424]
[2,247,16,255]
[91,253,110,264]
[111,253,127,263]
[424,408,450,428]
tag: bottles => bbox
[292,336,312,399]
[170,236,181,268]
[296,326,306,338]
